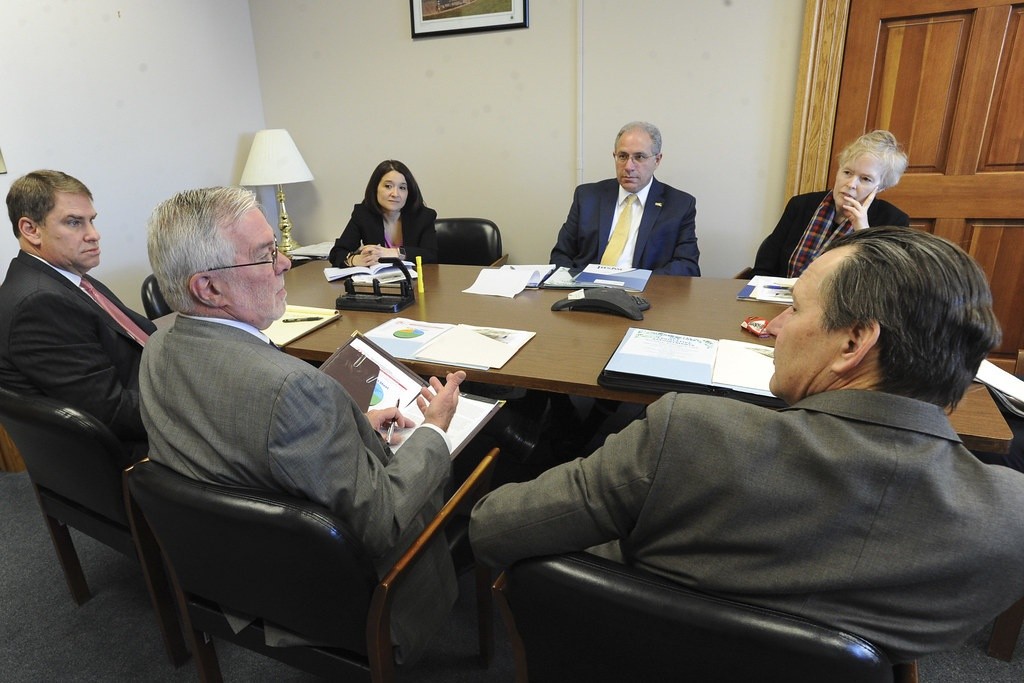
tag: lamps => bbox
[239,129,315,251]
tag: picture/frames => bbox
[409,0,530,39]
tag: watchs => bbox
[397,247,406,260]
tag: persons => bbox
[468,223,1024,667]
[329,160,437,269]
[138,188,547,663]
[749,131,910,277]
[549,122,701,277]
[0,170,157,444]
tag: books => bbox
[323,261,415,282]
[259,305,340,347]
[596,327,790,408]
[974,359,1024,417]
[365,317,536,371]
[317,331,507,462]
[464,265,653,300]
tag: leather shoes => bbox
[499,397,542,465]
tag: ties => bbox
[599,195,638,268]
[80,277,149,345]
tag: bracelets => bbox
[351,255,355,265]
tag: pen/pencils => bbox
[283,317,323,322]
[386,399,399,444]
[368,244,380,254]
[763,285,791,290]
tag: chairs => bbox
[0,384,188,669]
[142,276,173,319]
[433,219,503,267]
[128,443,513,683]
[490,551,919,682]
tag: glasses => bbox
[193,235,278,272]
[612,152,660,165]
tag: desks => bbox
[150,262,1015,454]
